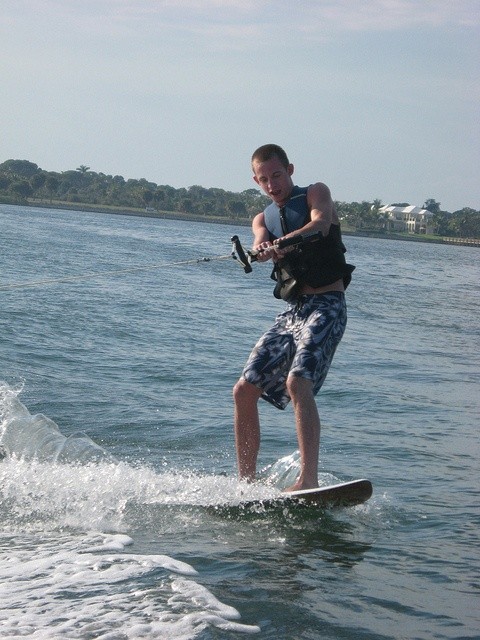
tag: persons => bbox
[233,144,347,493]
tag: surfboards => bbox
[279,479,373,509]
